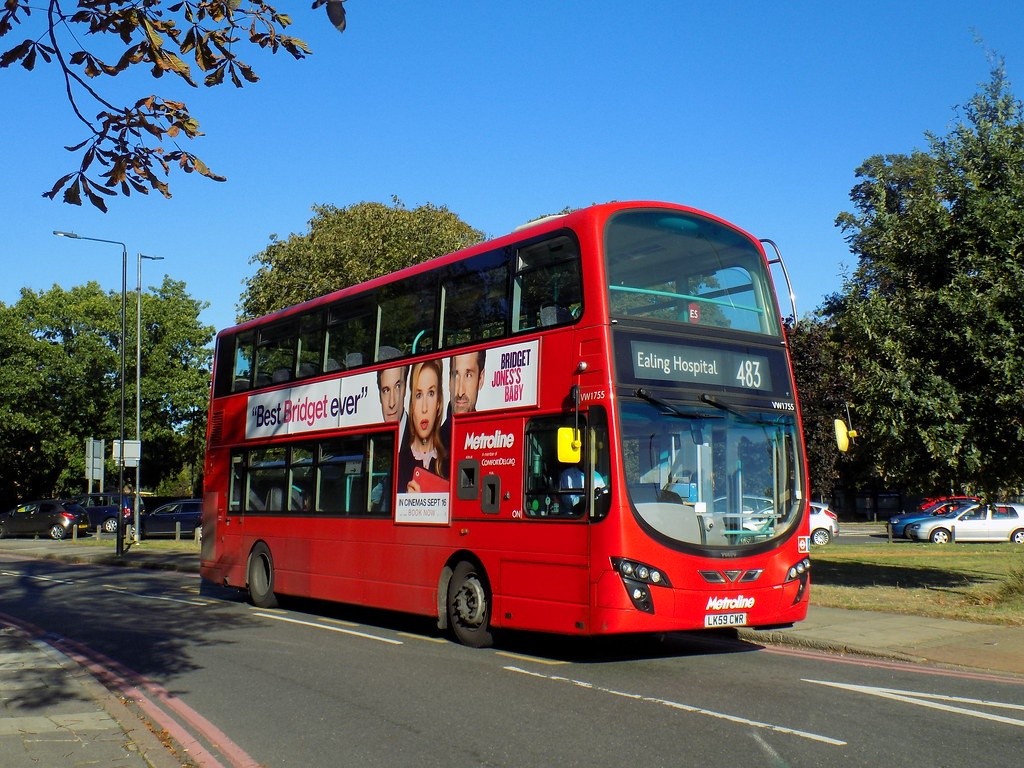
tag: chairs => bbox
[232,471,391,514]
[538,301,583,325]
[232,345,400,391]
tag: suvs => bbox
[70,492,146,532]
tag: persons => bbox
[946,505,953,516]
[298,473,312,512]
[968,510,980,520]
[377,348,486,494]
[371,475,386,512]
[558,446,606,515]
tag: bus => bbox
[198,201,858,648]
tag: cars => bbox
[54,230,127,559]
[125,498,203,540]
[910,502,1024,543]
[714,495,840,545]
[0,499,91,540]
[887,495,1008,539]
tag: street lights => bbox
[134,252,164,544]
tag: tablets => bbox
[412,467,450,494]
